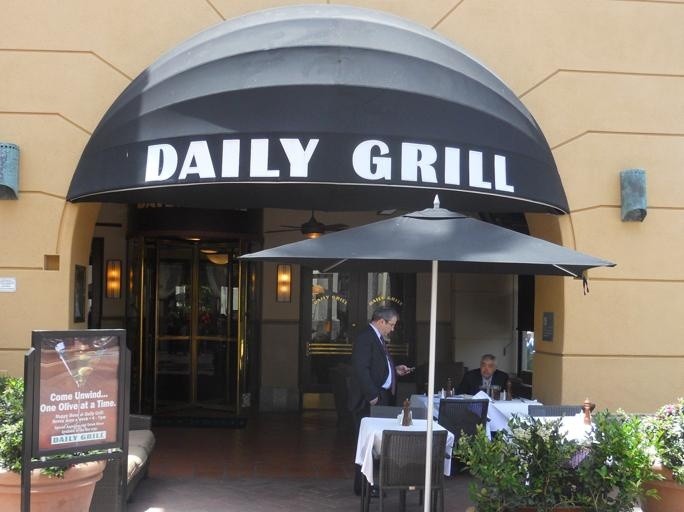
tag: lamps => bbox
[303,233,321,239]
[621,169,649,225]
[277,266,290,303]
[1,143,20,196]
[106,262,122,298]
[353,389,595,512]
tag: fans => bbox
[265,210,349,234]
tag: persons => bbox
[455,354,509,396]
[346,306,410,498]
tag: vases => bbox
[637,466,684,512]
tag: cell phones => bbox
[402,366,415,374]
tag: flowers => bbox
[643,399,684,480]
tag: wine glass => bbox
[46,336,110,440]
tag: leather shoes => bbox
[359,488,384,497]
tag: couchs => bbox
[89,413,155,511]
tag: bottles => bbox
[402,397,413,426]
[506,379,512,401]
[445,376,452,397]
[583,395,591,424]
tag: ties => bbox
[380,335,396,395]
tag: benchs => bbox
[510,370,532,400]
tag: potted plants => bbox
[0,376,110,511]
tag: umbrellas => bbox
[236,193,616,512]
